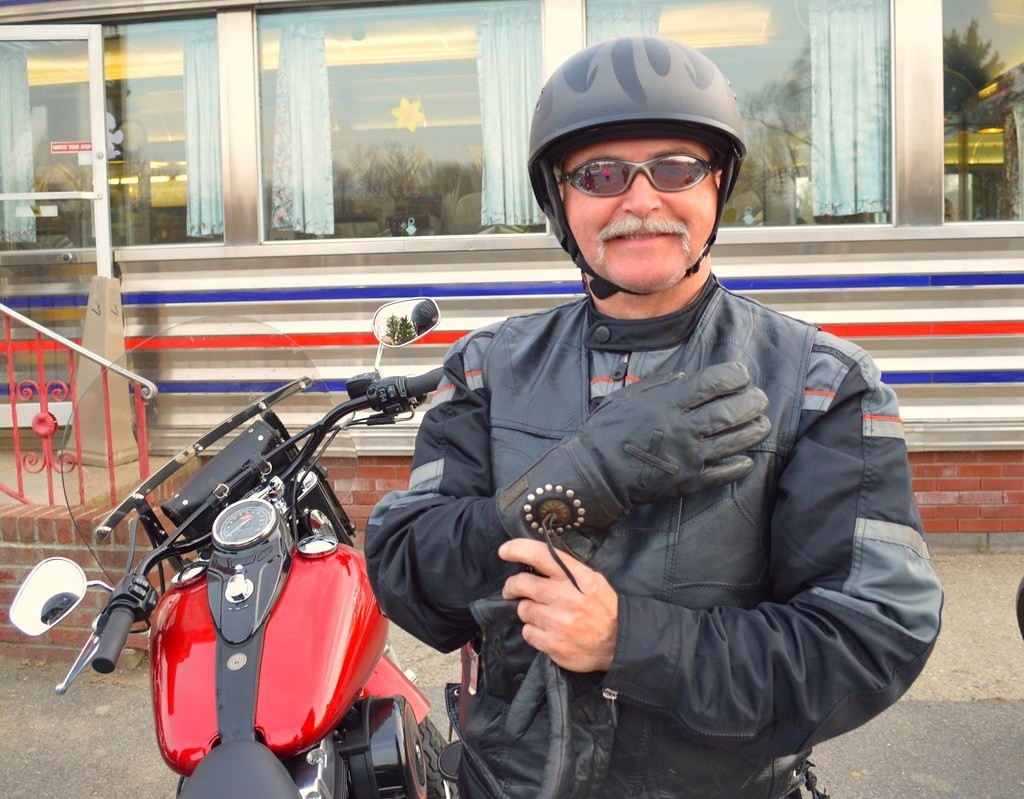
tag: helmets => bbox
[411,300,439,333]
[40,592,79,625]
[527,37,747,250]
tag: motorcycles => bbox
[8,295,462,799]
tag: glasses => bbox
[558,153,718,198]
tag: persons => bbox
[41,592,80,625]
[365,37,944,799]
[582,165,693,190]
[411,300,438,336]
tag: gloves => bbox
[494,362,772,552]
[469,599,613,799]
